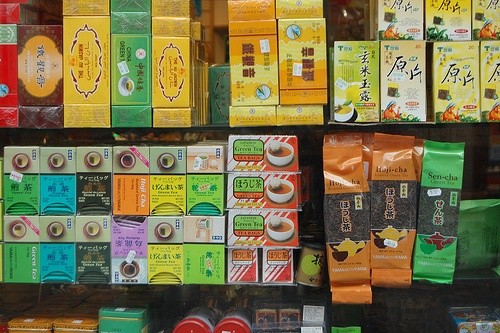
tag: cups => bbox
[50,223,63,236]
[171,306,218,333]
[15,155,28,168]
[123,264,136,277]
[87,153,100,166]
[213,305,252,333]
[12,223,25,236]
[158,224,172,238]
[86,222,99,236]
[160,154,174,168]
[50,154,64,167]
[197,219,211,229]
[121,153,135,168]
[198,154,208,169]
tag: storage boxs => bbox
[0,0,327,333]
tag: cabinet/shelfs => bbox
[0,122,500,294]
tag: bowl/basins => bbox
[266,142,294,166]
[118,76,134,97]
[267,217,294,241]
[266,179,294,204]
[334,100,354,122]
